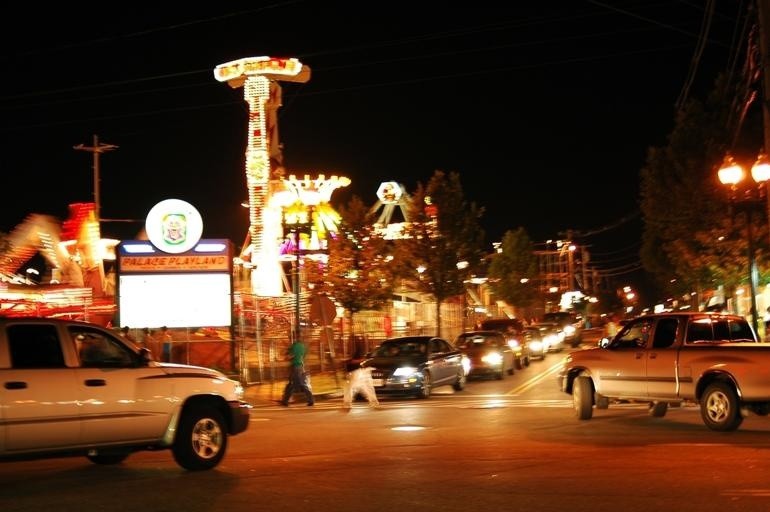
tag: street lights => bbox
[717,152,770,343]
[277,189,321,340]
[454,261,470,331]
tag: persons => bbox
[142,328,160,361]
[272,331,315,407]
[150,329,157,341]
[123,326,136,344]
[390,344,402,355]
[416,343,433,357]
[158,326,173,363]
[328,329,382,412]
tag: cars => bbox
[0,317,250,472]
[355,336,471,401]
[455,330,516,381]
[483,312,584,370]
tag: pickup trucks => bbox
[559,310,770,433]
